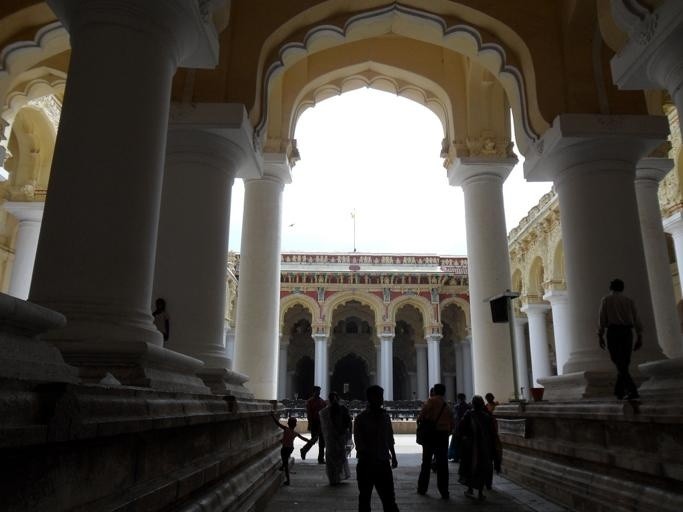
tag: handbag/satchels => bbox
[417,419,437,445]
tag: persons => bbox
[596,278,645,399]
[270,381,503,511]
[151,296,170,345]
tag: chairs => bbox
[277,398,424,421]
